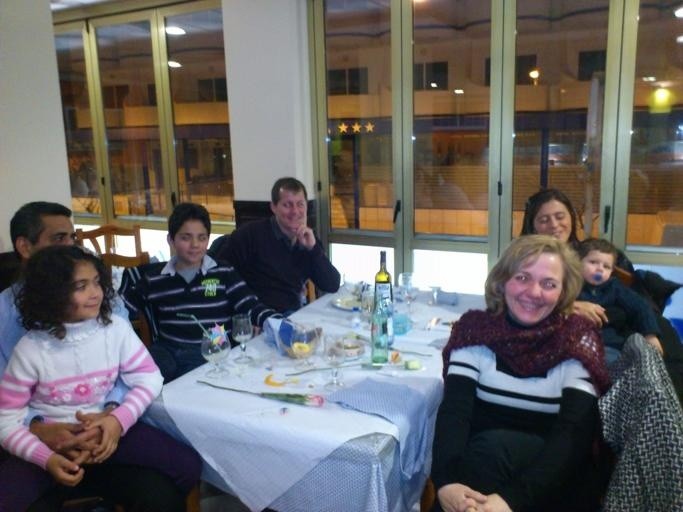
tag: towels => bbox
[325,376,432,482]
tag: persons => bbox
[573,236,665,389]
[210,176,340,337]
[1,200,187,512]
[519,187,609,328]
[1,244,203,512]
[116,202,286,377]
[427,233,613,512]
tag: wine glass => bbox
[343,272,419,330]
[201,314,347,393]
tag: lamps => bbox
[165,19,186,35]
[168,54,183,67]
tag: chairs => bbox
[232,199,317,304]
[95,251,153,347]
[597,333,682,511]
[74,224,142,258]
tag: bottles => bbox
[374,251,394,318]
[371,293,389,363]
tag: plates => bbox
[331,294,362,310]
